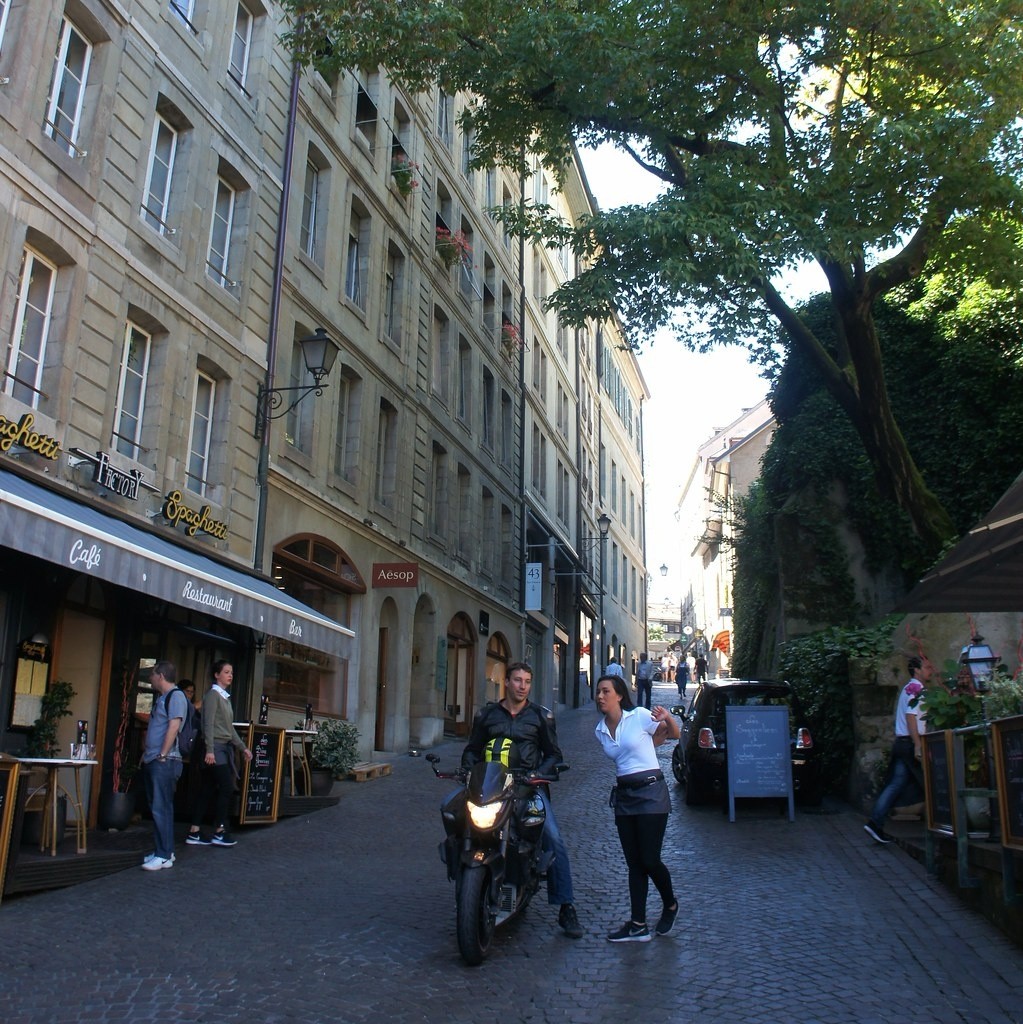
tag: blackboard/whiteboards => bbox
[240,723,287,824]
[725,705,794,797]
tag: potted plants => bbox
[294,718,364,797]
[11,681,78,848]
[98,729,140,833]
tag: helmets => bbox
[481,737,519,775]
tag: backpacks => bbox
[164,689,195,758]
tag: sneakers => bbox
[210,830,238,846]
[655,900,680,936]
[186,831,211,845]
[606,921,652,942]
[558,903,583,938]
[864,821,892,843]
[141,857,173,870]
[143,852,176,863]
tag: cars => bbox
[670,678,826,810]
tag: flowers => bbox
[393,155,419,198]
[504,320,525,356]
[435,227,478,268]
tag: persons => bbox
[675,655,690,699]
[864,657,933,843]
[441,663,584,939]
[176,679,198,780]
[661,652,679,684]
[605,657,623,678]
[686,652,697,684]
[185,661,253,845]
[635,653,653,711]
[141,662,188,871]
[594,675,680,943]
[695,654,709,685]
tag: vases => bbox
[502,343,510,356]
[436,250,446,266]
[391,175,399,192]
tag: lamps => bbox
[583,512,612,552]
[956,630,1001,695]
[254,326,343,441]
[250,630,270,654]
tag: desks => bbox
[15,757,99,857]
[233,722,319,797]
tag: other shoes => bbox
[680,695,682,699]
[683,692,686,697]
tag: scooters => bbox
[420,751,574,964]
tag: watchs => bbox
[158,754,166,759]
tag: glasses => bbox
[185,689,194,695]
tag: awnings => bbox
[0,469,356,660]
